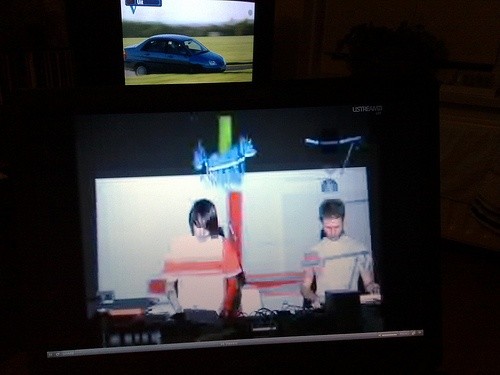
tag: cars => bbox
[124,33,226,76]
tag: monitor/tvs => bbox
[120,0,255,85]
[46,105,424,357]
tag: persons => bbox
[299,198,381,310]
[162,199,243,325]
